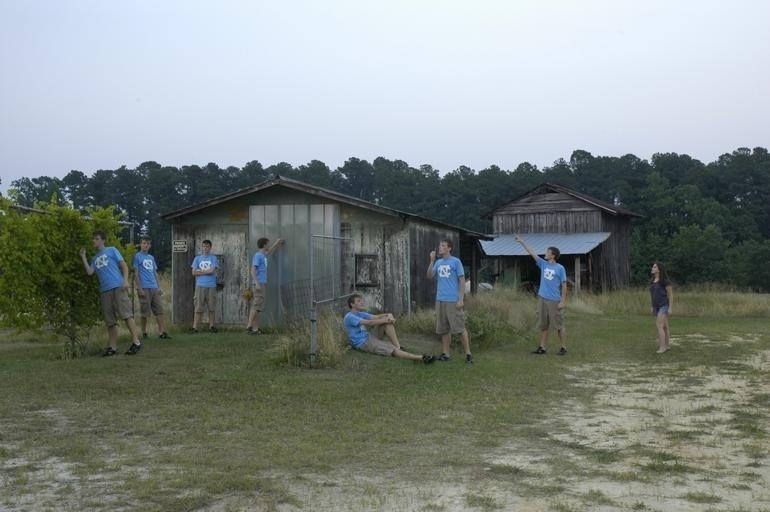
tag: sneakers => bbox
[125,343,144,355]
[538,347,545,353]
[422,353,472,364]
[103,350,117,356]
[160,332,171,339]
[560,347,567,355]
[190,326,265,335]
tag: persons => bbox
[132,232,173,340]
[425,238,473,365]
[189,238,220,335]
[647,260,675,355]
[511,230,568,356]
[246,236,286,337]
[342,293,436,364]
[77,229,141,358]
